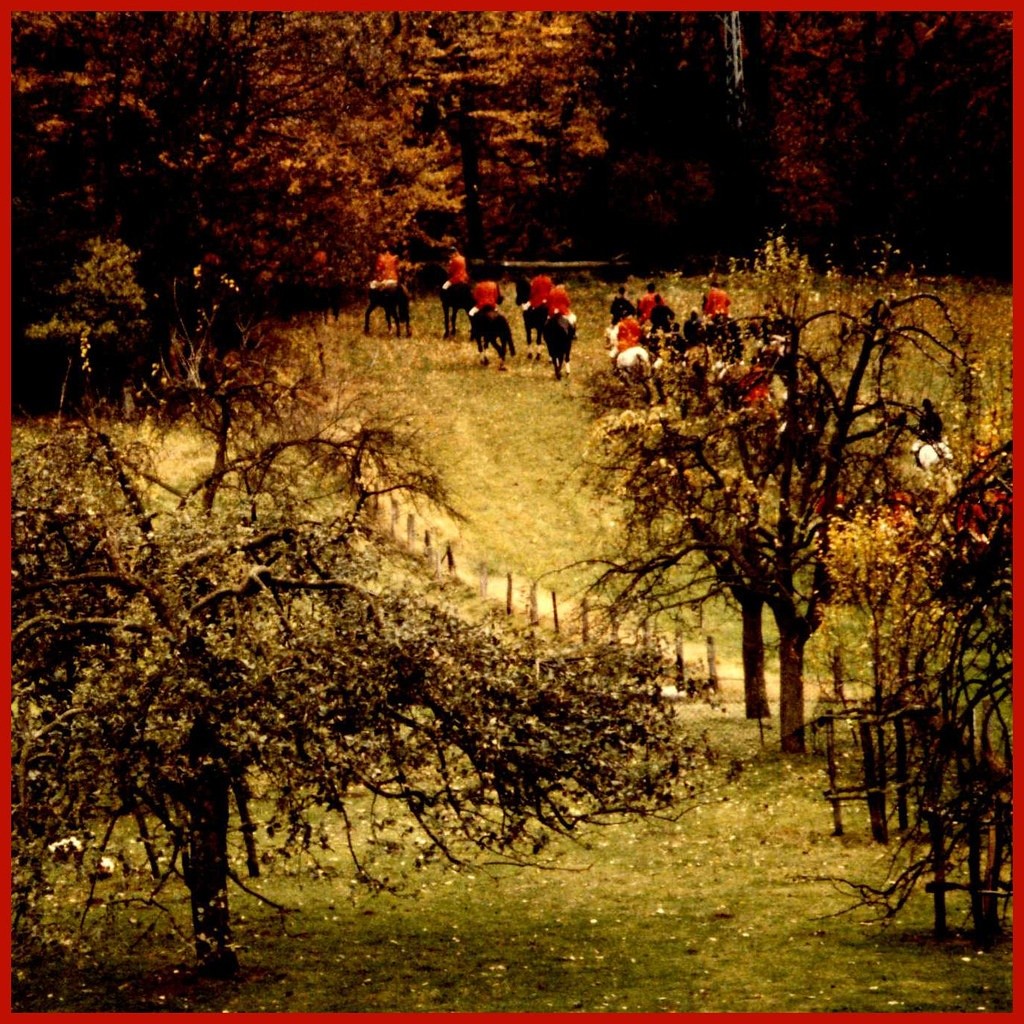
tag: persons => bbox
[813,480,1013,545]
[438,246,468,311]
[468,272,498,341]
[522,265,577,340]
[604,281,793,427]
[912,398,943,467]
[370,243,399,307]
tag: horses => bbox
[363,285,955,475]
